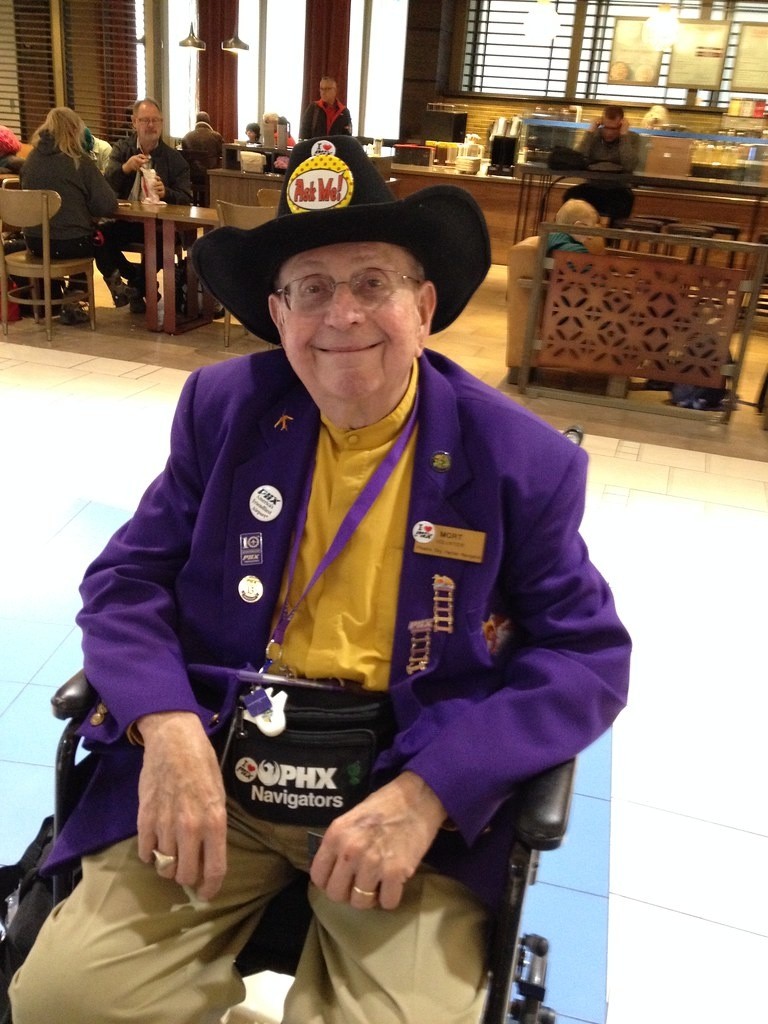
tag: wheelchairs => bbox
[1,423,584,1024]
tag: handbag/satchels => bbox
[206,686,394,829]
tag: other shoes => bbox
[130,292,161,313]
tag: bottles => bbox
[715,128,746,146]
[277,116,290,151]
[758,130,768,161]
[374,139,382,156]
[727,97,766,118]
[693,140,714,162]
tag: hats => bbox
[191,136,492,347]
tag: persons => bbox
[0,96,194,324]
[4,136,631,1024]
[181,111,223,205]
[575,106,641,173]
[245,123,261,143]
[264,113,295,147]
[298,75,353,137]
[544,199,608,255]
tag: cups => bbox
[447,142,458,162]
[263,123,274,147]
[437,142,447,165]
[426,140,437,165]
[714,146,744,165]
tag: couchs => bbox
[506,235,543,384]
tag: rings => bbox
[153,850,176,867]
[353,887,379,896]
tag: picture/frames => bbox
[607,16,664,88]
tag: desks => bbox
[692,159,768,183]
[109,199,219,335]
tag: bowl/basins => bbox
[609,61,628,81]
[655,124,687,133]
[237,140,247,145]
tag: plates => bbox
[634,63,656,82]
[456,156,481,171]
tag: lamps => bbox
[179,22,206,51]
[221,0,248,52]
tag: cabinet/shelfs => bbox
[207,143,292,206]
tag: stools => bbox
[612,214,768,319]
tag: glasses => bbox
[277,270,422,304]
[135,116,161,123]
[319,86,335,92]
[602,122,621,130]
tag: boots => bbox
[103,269,138,309]
[60,288,89,324]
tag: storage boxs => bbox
[392,144,435,166]
[636,133,695,177]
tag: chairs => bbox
[0,188,95,341]
[216,188,281,347]
